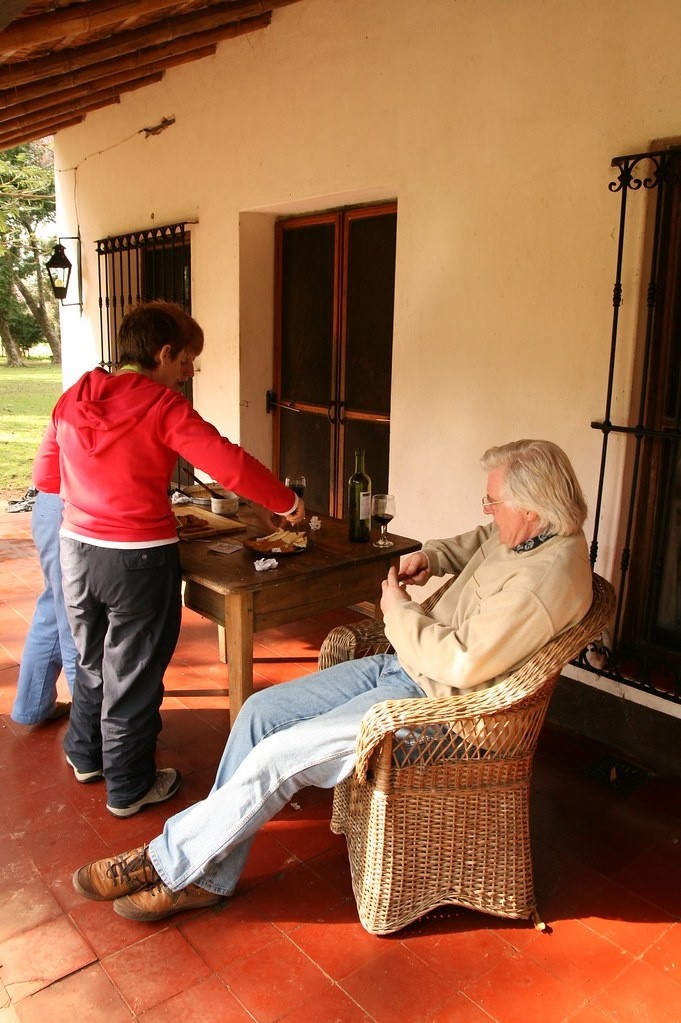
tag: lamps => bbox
[44,226,83,314]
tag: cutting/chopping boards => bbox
[171,505,248,542]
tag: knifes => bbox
[290,521,300,537]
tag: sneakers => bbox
[106,767,182,819]
[113,880,222,922]
[72,842,161,901]
[66,754,103,783]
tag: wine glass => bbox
[372,493,395,548]
[284,476,308,520]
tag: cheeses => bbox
[267,530,307,548]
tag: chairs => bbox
[318,571,618,936]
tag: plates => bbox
[182,482,226,505]
[243,532,315,558]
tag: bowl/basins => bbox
[210,493,240,514]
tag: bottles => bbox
[348,449,372,543]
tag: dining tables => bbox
[174,484,423,730]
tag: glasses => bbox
[481,496,505,511]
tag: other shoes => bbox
[48,701,72,719]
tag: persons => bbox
[72,440,591,921]
[57,301,306,816]
[11,312,204,725]
